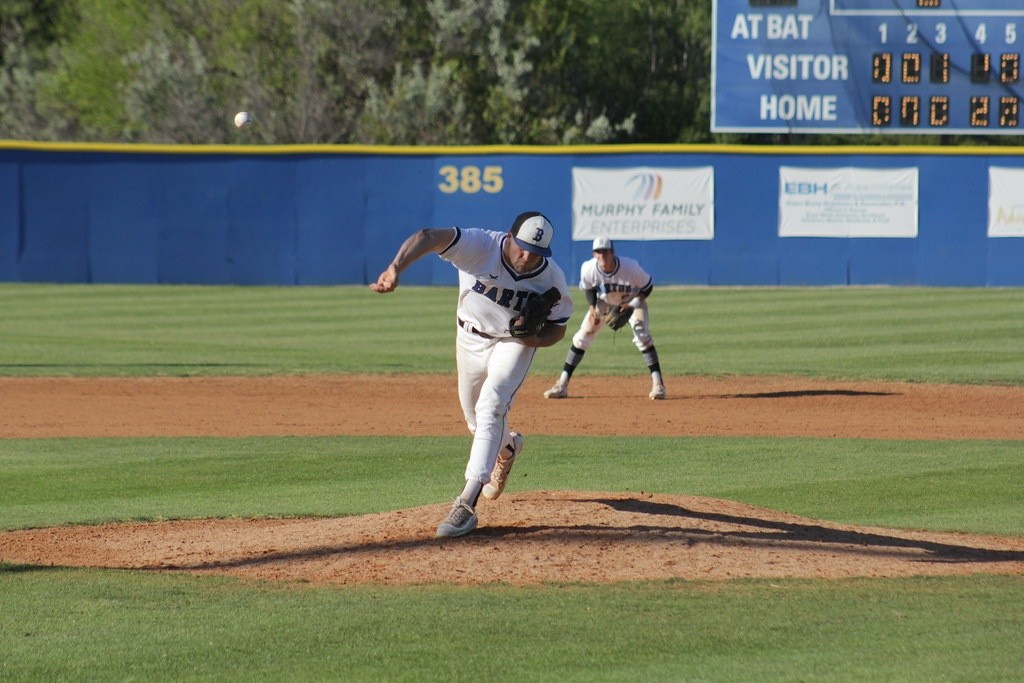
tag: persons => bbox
[368,211,573,537]
[543,236,665,399]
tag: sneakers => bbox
[482,432,524,500]
[543,380,568,399]
[436,496,478,537]
[648,383,666,400]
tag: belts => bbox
[458,317,496,340]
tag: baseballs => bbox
[233,111,254,131]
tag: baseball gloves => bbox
[508,286,562,339]
[605,306,634,331]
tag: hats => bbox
[510,211,554,257]
[592,236,613,251]
